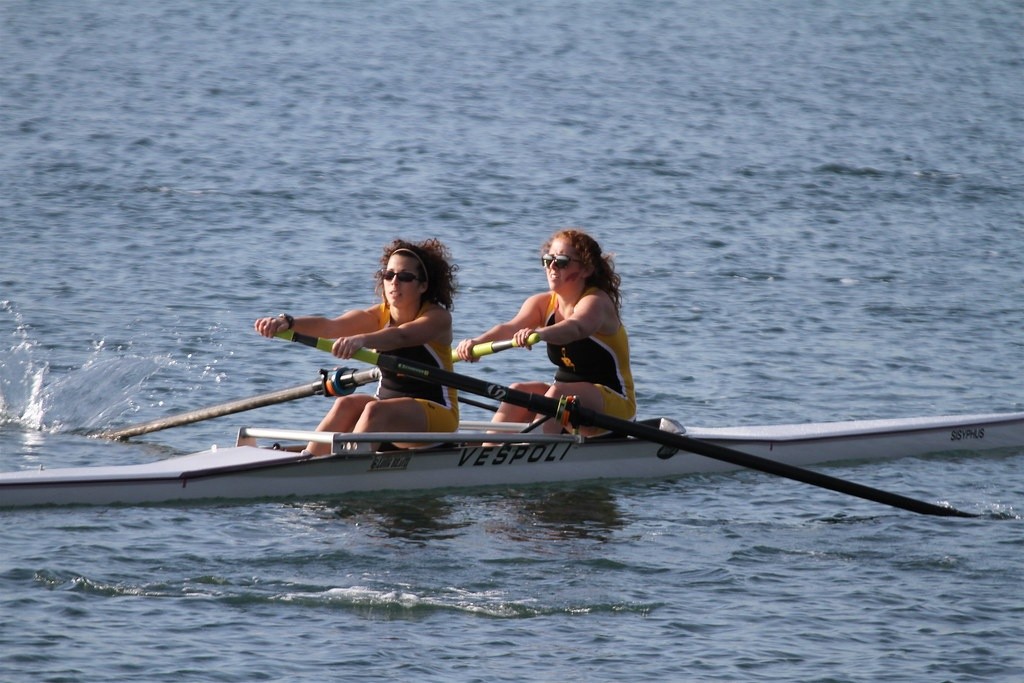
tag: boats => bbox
[0,410,1024,511]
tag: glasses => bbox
[381,269,419,282]
[542,254,583,268]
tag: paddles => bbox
[88,330,979,519]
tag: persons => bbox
[254,237,460,458]
[456,229,639,447]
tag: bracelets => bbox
[279,313,295,330]
